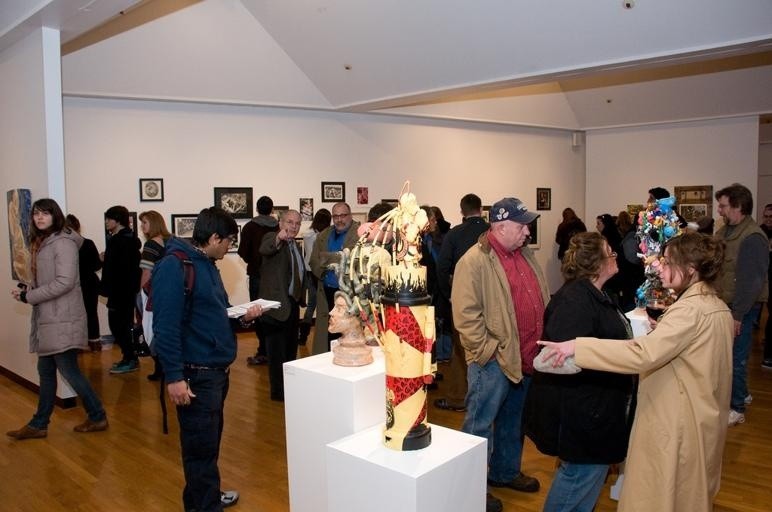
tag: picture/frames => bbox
[104,178,399,254]
[626,202,644,224]
[479,187,552,249]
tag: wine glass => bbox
[645,298,665,322]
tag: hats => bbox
[489,197,541,224]
[105,206,129,227]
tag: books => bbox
[226,297,282,320]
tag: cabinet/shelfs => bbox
[282,344,489,512]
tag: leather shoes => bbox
[435,399,465,412]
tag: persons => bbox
[328,292,373,367]
[357,193,492,411]
[698,186,772,423]
[238,197,329,402]
[519,229,641,512]
[65,212,106,353]
[553,187,687,310]
[138,210,173,382]
[534,230,736,511]
[308,202,360,353]
[450,197,553,512]
[3,197,108,440]
[98,204,143,375]
[136,205,266,512]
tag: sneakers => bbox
[7,425,47,438]
[220,490,239,508]
[89,341,102,351]
[110,350,161,380]
[744,394,753,403]
[427,358,451,389]
[761,361,772,369]
[727,409,745,427]
[487,492,503,511]
[247,355,268,365]
[487,473,540,493]
[74,420,109,432]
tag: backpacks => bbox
[140,249,195,347]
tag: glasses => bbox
[610,252,618,257]
[333,214,347,218]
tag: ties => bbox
[292,249,301,302]
